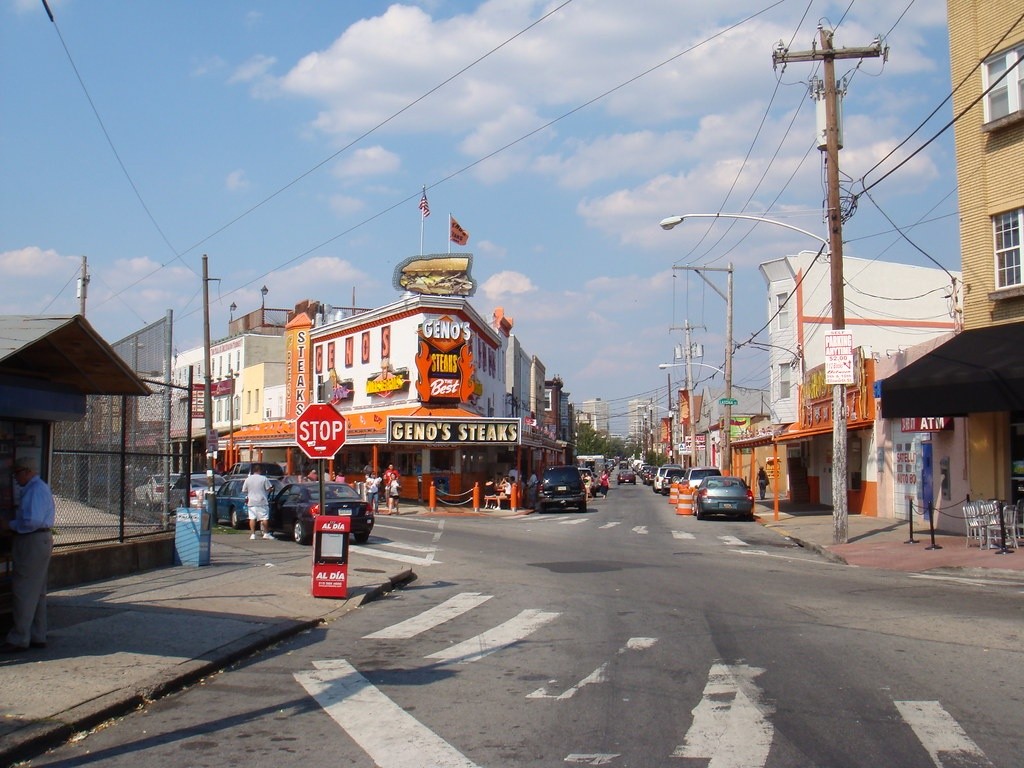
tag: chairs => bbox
[963,498,1024,550]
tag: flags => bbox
[419,188,430,217]
[449,217,469,246]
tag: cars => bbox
[265,481,376,545]
[135,473,184,512]
[682,467,722,490]
[628,458,660,486]
[692,476,755,519]
[605,459,617,478]
[215,478,290,530]
[167,474,227,510]
[616,470,639,485]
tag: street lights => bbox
[658,363,743,483]
[230,302,238,323]
[634,420,658,465]
[260,285,269,326]
[637,404,675,465]
[661,214,859,548]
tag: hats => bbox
[759,466,764,470]
[9,456,37,471]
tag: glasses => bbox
[9,468,25,478]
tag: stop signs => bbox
[293,403,348,462]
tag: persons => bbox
[756,467,769,500]
[363,461,402,515]
[0,457,55,652]
[483,476,516,510]
[580,473,591,501]
[508,463,518,481]
[599,470,610,499]
[335,470,345,482]
[242,464,276,540]
[527,471,537,509]
[306,468,330,481]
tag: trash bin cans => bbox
[311,516,351,599]
[174,507,211,567]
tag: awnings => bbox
[881,321,1024,417]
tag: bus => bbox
[577,455,606,492]
[619,461,629,470]
[222,461,285,482]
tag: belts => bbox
[29,528,50,533]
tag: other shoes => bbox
[494,506,501,510]
[375,512,380,515]
[29,641,47,648]
[0,643,28,653]
[250,533,257,540]
[262,532,275,540]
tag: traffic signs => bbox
[822,329,856,385]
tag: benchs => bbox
[484,497,511,508]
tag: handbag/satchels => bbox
[765,477,770,486]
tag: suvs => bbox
[577,466,598,498]
[653,467,682,494]
[537,465,587,512]
[660,467,686,496]
